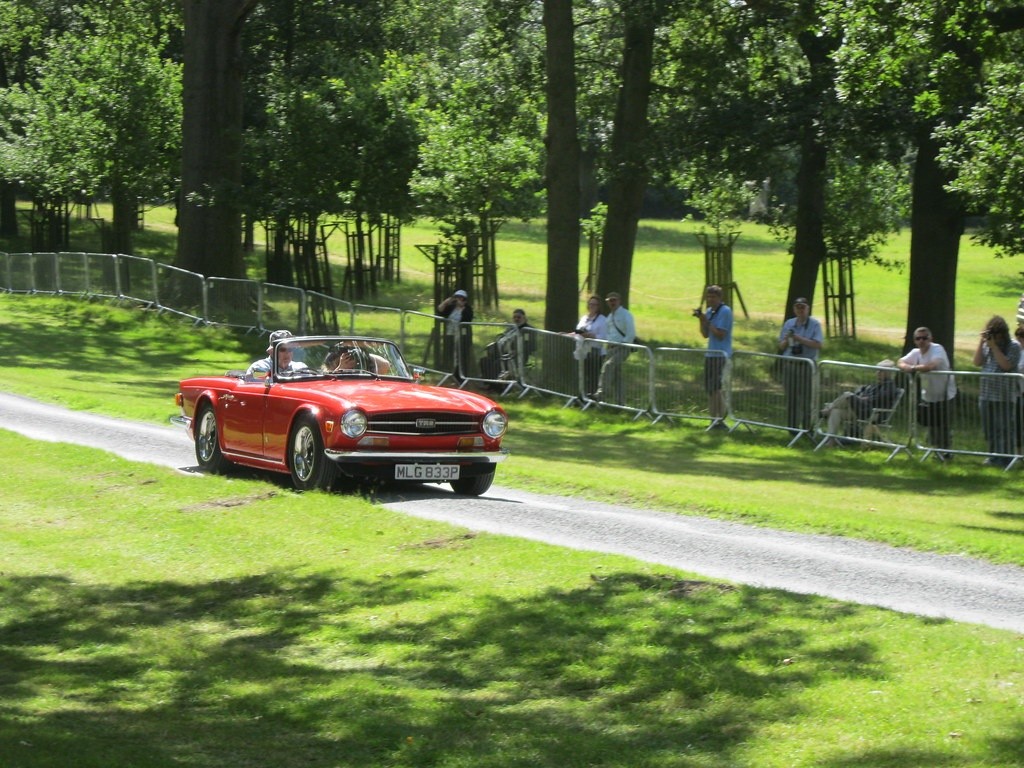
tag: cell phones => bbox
[575,330,583,334]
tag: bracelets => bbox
[707,321,711,325]
[912,365,916,371]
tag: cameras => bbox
[348,349,363,361]
[692,309,702,317]
[984,329,994,339]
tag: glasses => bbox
[279,347,293,353]
[915,336,930,341]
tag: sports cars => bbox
[170,336,508,496]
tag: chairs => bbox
[823,387,906,451]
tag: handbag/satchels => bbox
[630,339,639,353]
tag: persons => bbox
[1015,326,1024,459]
[321,340,389,375]
[481,309,534,379]
[973,316,1020,467]
[570,296,607,397]
[593,292,635,413]
[244,330,307,375]
[693,286,733,428]
[898,327,957,460]
[438,290,473,375]
[778,298,822,438]
[819,359,896,448]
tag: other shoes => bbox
[825,437,840,447]
[932,451,954,462]
[712,420,729,430]
[788,426,801,434]
[802,428,815,436]
[818,409,828,419]
[982,456,1000,464]
[591,391,608,401]
[614,405,626,414]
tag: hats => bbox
[453,290,468,298]
[265,330,296,354]
[792,297,811,308]
[876,358,895,367]
[605,291,622,302]
